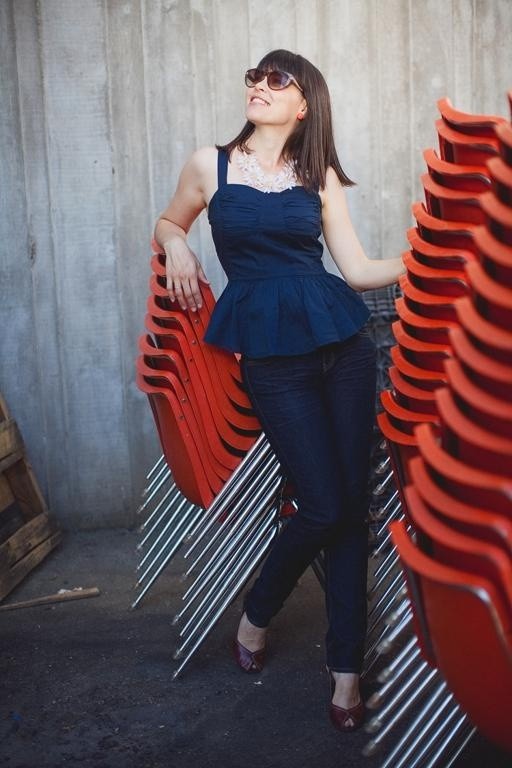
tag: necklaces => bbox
[234,145,298,195]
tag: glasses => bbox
[246,68,306,93]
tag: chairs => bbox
[131,94,512,768]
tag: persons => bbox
[154,49,407,733]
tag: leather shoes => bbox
[233,592,266,674]
[325,664,364,732]
